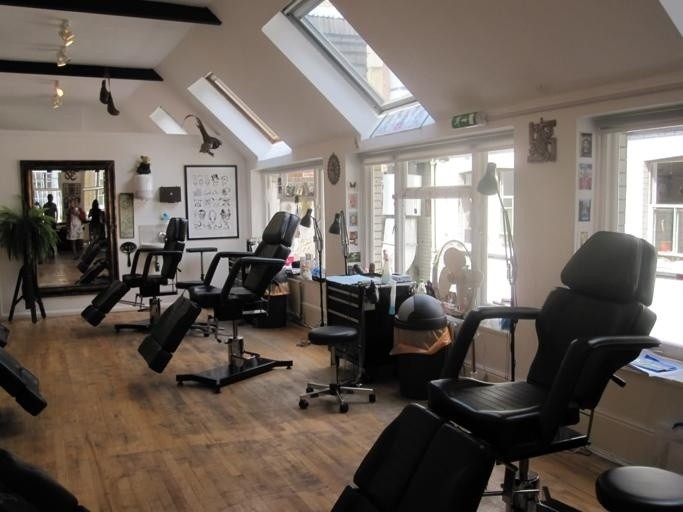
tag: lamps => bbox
[181,114,222,157]
[34,201,43,218]
[99,67,119,116]
[133,174,154,200]
[301,208,324,327]
[327,210,349,275]
[50,17,74,110]
[477,163,517,383]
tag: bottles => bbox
[416,279,426,295]
[380,254,392,285]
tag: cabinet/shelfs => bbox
[320,273,417,382]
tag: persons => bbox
[43,194,58,229]
[66,197,85,260]
[88,199,103,234]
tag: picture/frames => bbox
[119,193,134,239]
[576,128,596,255]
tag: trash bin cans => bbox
[392,294,450,399]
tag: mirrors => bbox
[20,160,119,298]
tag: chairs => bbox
[74,236,111,285]
[137,212,299,394]
[0,347,48,418]
[0,447,89,512]
[331,231,661,512]
[80,217,218,337]
[0,323,7,345]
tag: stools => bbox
[175,280,216,337]
[186,247,217,281]
[299,325,375,413]
[594,464,683,512]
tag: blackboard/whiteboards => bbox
[62,182,80,209]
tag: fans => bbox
[432,239,488,382]
